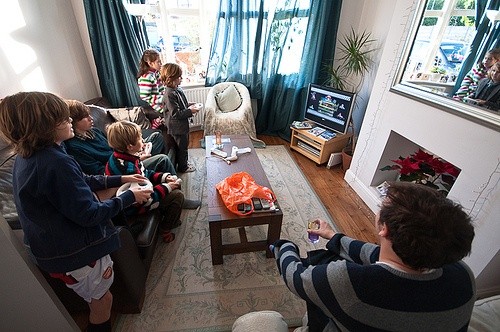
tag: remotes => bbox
[260,199,270,209]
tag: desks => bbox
[204,133,283,266]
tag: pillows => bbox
[214,84,244,113]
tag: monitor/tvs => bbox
[303,82,357,136]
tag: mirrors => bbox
[388,0,500,134]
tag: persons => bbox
[136,50,167,114]
[105,120,184,242]
[452,48,500,101]
[467,62,500,112]
[160,63,200,172]
[63,98,202,210]
[269,181,476,331]
[1,92,155,331]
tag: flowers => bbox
[381,147,461,191]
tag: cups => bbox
[216,130,221,145]
[307,217,321,245]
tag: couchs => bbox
[0,96,168,316]
[204,81,257,149]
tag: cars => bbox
[154,35,191,51]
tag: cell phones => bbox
[251,198,262,210]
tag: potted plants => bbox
[320,24,382,172]
[440,69,449,82]
[429,66,447,81]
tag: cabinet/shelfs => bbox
[288,120,354,166]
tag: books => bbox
[318,129,337,141]
[307,126,326,137]
[291,120,312,130]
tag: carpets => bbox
[112,143,341,331]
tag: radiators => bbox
[182,86,213,132]
